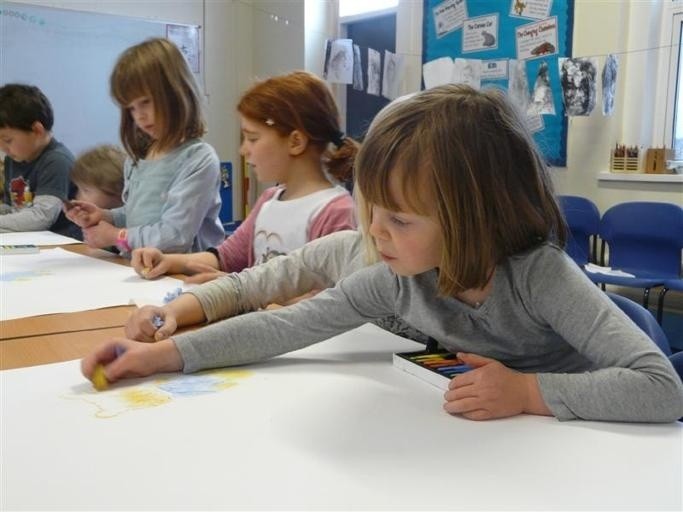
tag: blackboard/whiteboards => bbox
[0,0,204,161]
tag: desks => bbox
[0,294,681,506]
[0,228,315,339]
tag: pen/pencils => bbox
[54,193,74,210]
[409,352,475,379]
[613,142,625,157]
[626,144,643,158]
[91,362,107,391]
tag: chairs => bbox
[550,195,602,292]
[656,277,683,324]
[604,291,681,379]
[584,201,683,309]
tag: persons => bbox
[60,36,228,262]
[533,61,552,112]
[0,82,82,244]
[127,69,357,285]
[68,143,124,214]
[559,58,596,116]
[79,82,681,423]
